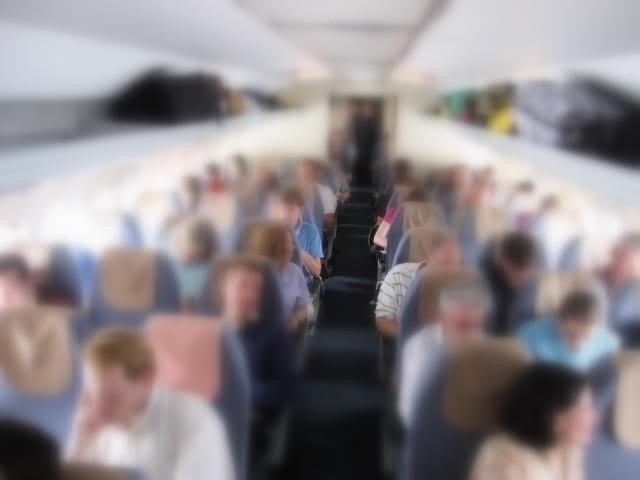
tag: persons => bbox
[0,137,640,479]
